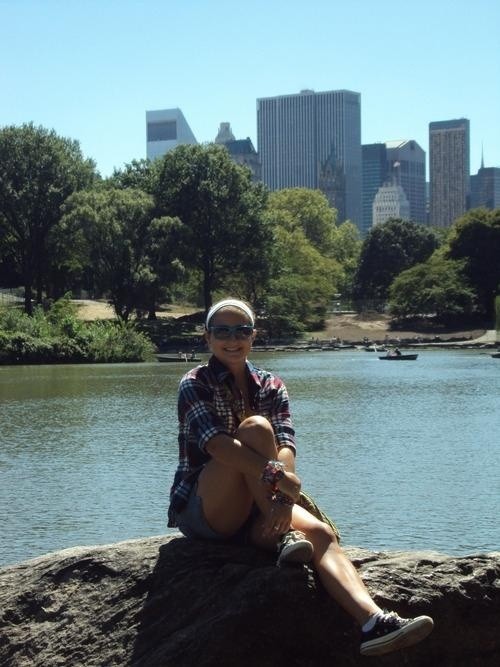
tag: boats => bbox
[279,341,390,352]
[490,351,500,358]
[155,355,202,364]
[378,352,420,360]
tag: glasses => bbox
[207,322,256,342]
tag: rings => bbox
[272,526,279,530]
[295,489,299,496]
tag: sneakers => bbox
[356,615,438,659]
[275,530,314,577]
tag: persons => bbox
[191,350,196,362]
[178,352,182,358]
[331,335,344,345]
[386,348,392,357]
[307,336,320,346]
[165,294,434,655]
[394,347,401,356]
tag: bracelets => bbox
[260,459,286,487]
[270,489,294,507]
